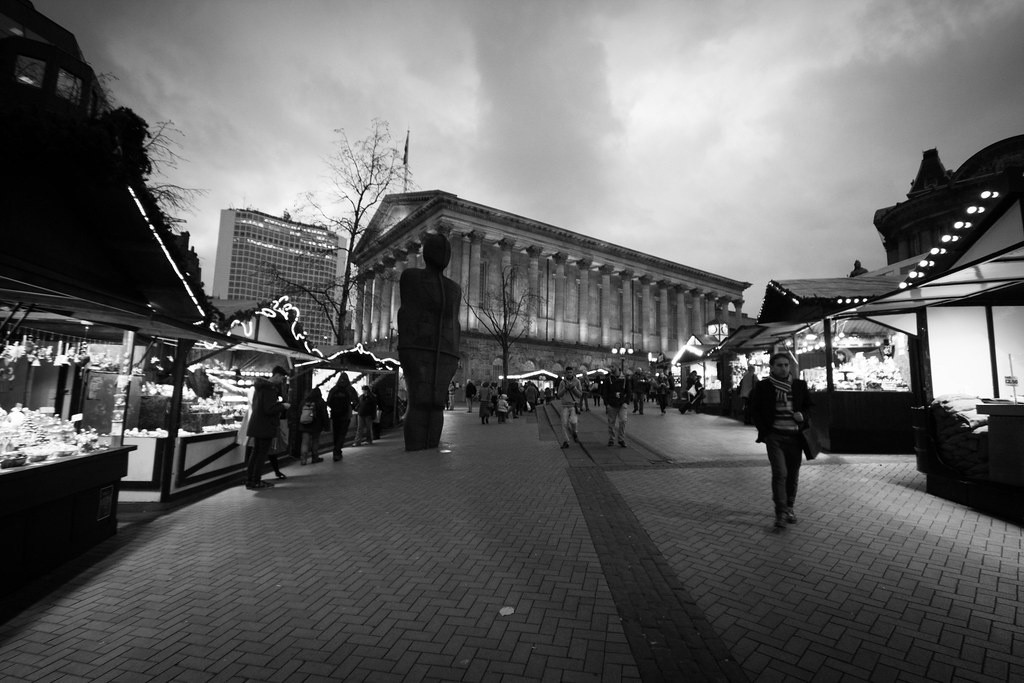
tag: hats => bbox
[500,394,508,399]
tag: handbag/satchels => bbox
[574,401,582,414]
[526,401,532,411]
[300,401,315,424]
[688,385,697,396]
[800,425,821,460]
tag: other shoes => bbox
[662,409,665,413]
[301,456,306,465]
[618,441,628,447]
[639,412,643,414]
[312,458,323,463]
[480,412,522,424]
[608,440,614,446]
[247,481,274,489]
[560,442,569,449]
[333,454,343,461]
[573,432,577,440]
[786,506,798,523]
[632,409,637,413]
[352,442,361,447]
[773,508,788,528]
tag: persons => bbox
[631,367,649,415]
[327,372,359,462]
[235,365,291,489]
[479,380,561,425]
[601,361,629,448]
[466,379,477,413]
[298,387,332,466]
[352,380,383,447]
[396,233,462,453]
[443,381,455,411]
[748,354,810,528]
[579,370,705,425]
[739,365,759,426]
[850,259,867,277]
[558,367,582,449]
[834,348,854,363]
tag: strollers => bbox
[678,387,706,415]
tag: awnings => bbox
[31,309,240,374]
[757,273,909,337]
[312,362,396,389]
[831,239,1024,336]
[718,324,816,365]
[154,334,332,382]
[0,257,150,378]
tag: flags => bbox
[403,135,408,163]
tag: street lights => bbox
[610,341,635,373]
